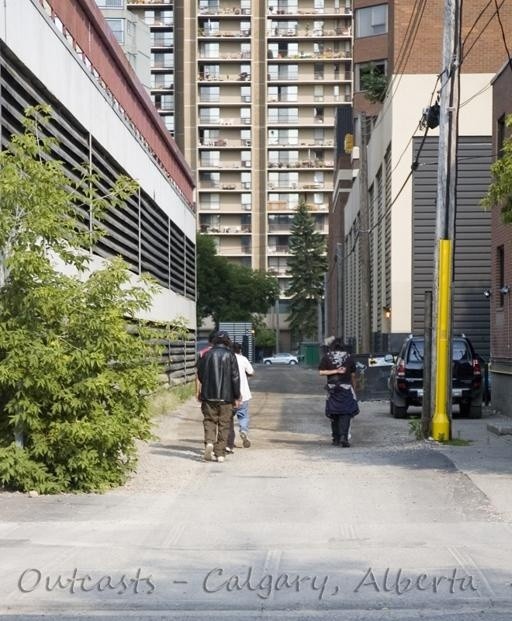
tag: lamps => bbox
[383,306,391,319]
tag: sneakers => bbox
[204,429,251,462]
[331,439,350,447]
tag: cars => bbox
[195,340,210,358]
[262,350,300,366]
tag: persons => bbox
[232,343,255,449]
[318,337,356,448]
[196,333,217,403]
[199,330,239,462]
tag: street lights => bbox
[269,265,281,352]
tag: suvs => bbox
[382,329,487,420]
